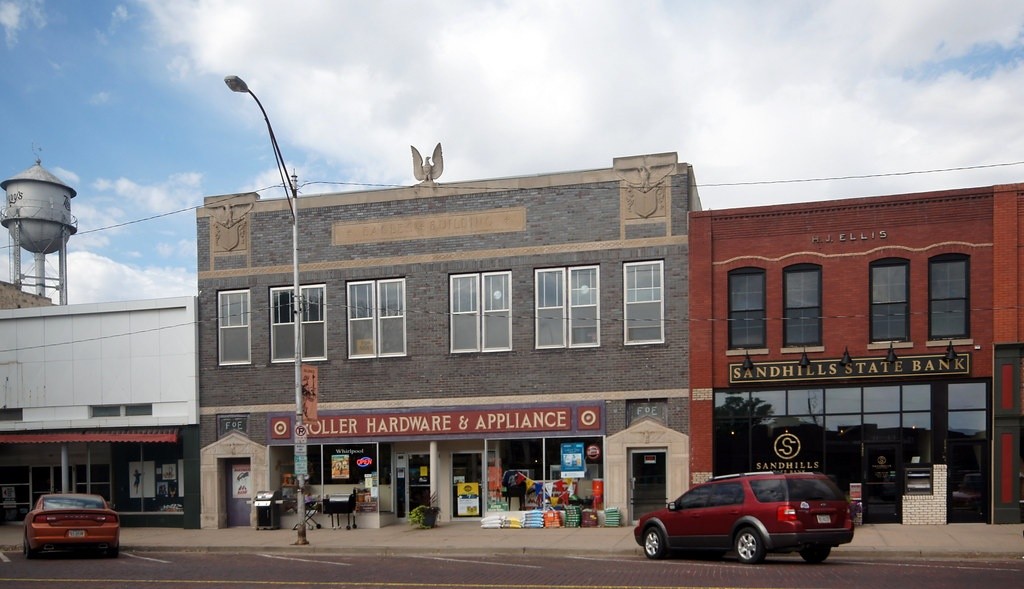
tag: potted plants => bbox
[409,505,440,530]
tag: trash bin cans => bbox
[252,490,280,530]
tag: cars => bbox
[632,468,855,566]
[21,494,123,557]
[951,474,986,514]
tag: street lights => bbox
[223,74,312,546]
